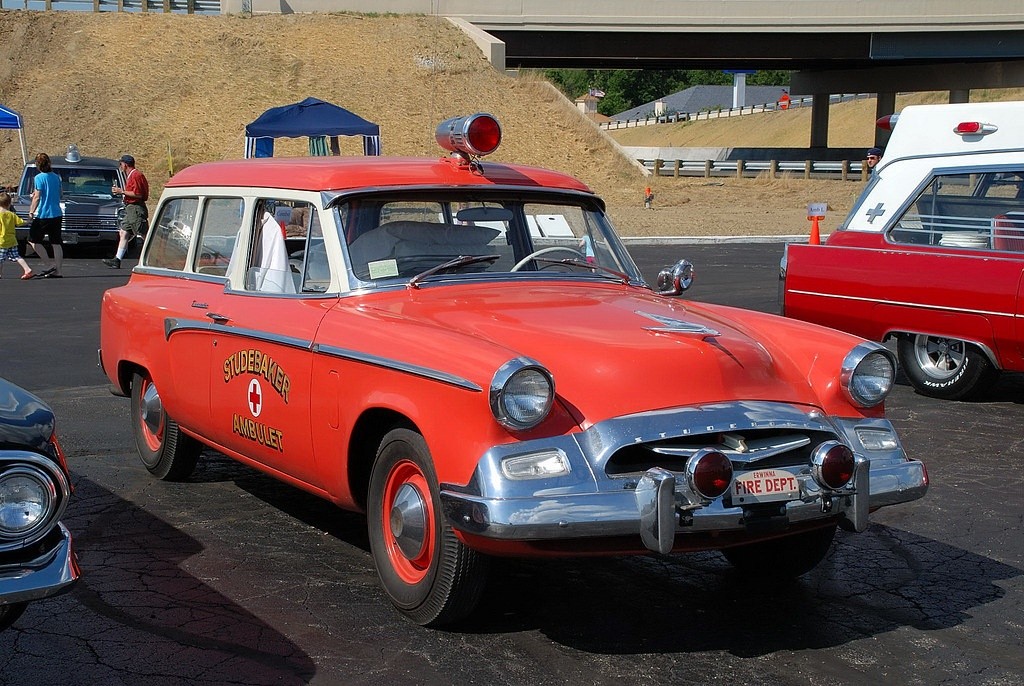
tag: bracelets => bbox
[30,213,33,214]
[122,190,125,194]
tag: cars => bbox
[657,110,689,122]
[780,100,1024,400]
[5,143,137,256]
[96,111,929,627]
[0,379,80,632]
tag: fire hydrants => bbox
[643,188,654,209]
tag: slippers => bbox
[38,267,64,278]
[21,274,36,280]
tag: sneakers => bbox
[102,259,120,269]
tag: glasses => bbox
[865,156,875,160]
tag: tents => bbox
[0,104,28,170]
[238,97,380,217]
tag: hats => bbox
[119,154,135,164]
[866,148,882,156]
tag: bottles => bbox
[113,180,118,195]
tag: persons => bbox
[579,225,603,272]
[0,192,32,279]
[102,155,149,269]
[867,148,883,168]
[29,153,63,278]
